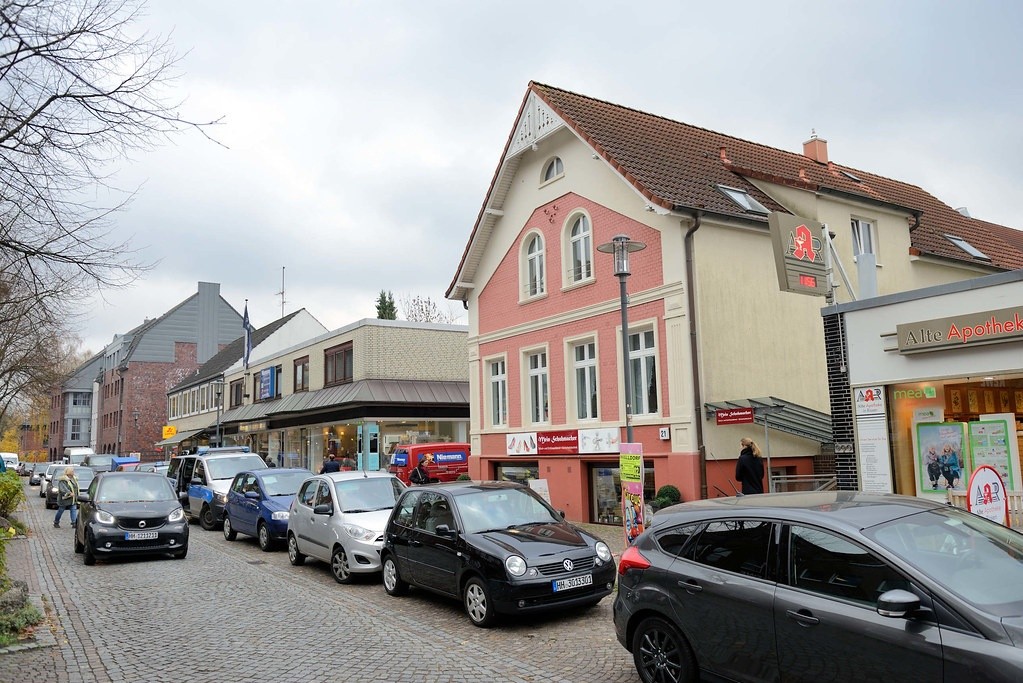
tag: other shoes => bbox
[946,484,955,490]
[54,520,60,528]
[933,484,938,490]
[71,521,76,528]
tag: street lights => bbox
[596,235,648,444]
[21,418,30,452]
[210,379,228,446]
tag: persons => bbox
[735,436,765,495]
[318,453,340,475]
[264,455,276,468]
[53,466,80,529]
[408,456,440,486]
[19,463,26,476]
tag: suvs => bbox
[80,455,119,473]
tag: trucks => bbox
[111,457,140,472]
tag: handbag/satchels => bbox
[410,481,422,486]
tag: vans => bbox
[62,448,95,465]
[0,453,19,471]
[389,441,473,486]
[168,446,268,533]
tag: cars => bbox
[44,467,96,509]
[74,472,189,566]
[17,463,27,473]
[223,469,329,553]
[40,464,81,497]
[29,462,53,486]
[617,491,1023,683]
[149,466,169,474]
[381,480,615,629]
[21,463,34,475]
[136,462,163,472]
[288,469,417,585]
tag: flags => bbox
[242,304,252,368]
